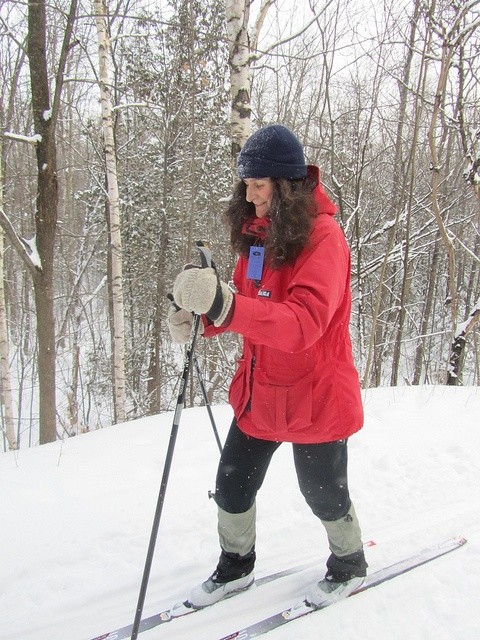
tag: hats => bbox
[235,125,308,180]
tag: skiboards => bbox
[92,535,471,640]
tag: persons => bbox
[165,124,369,609]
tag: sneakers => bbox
[187,571,254,606]
[305,576,366,609]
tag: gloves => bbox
[173,263,234,328]
[168,307,204,345]
